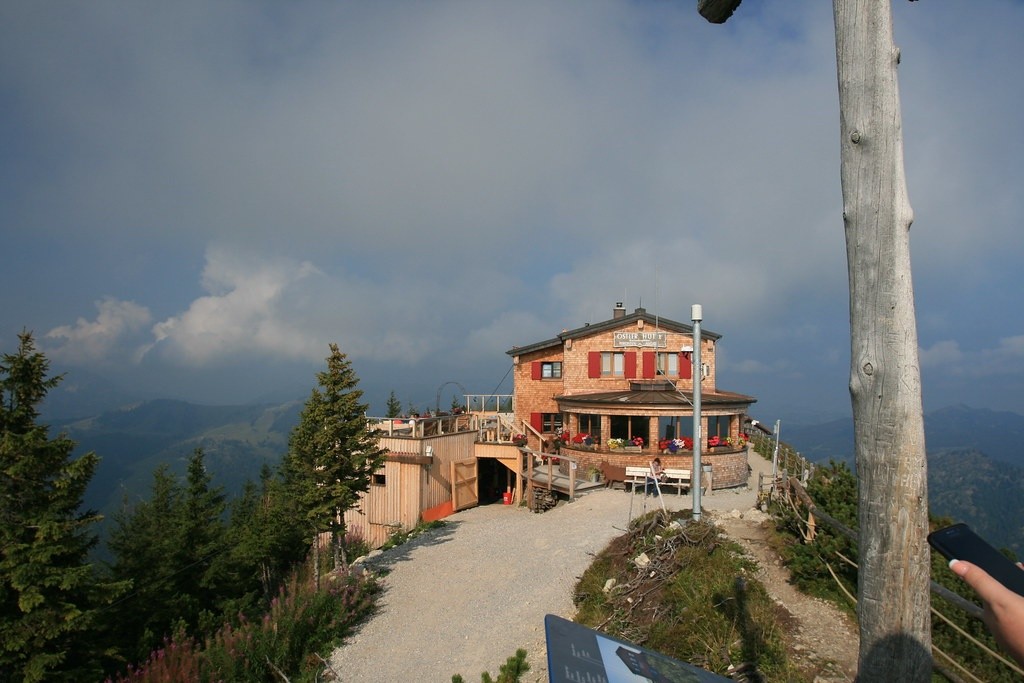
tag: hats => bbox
[654,458,660,463]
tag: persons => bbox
[393,413,421,437]
[949,558,1024,683]
[646,457,665,498]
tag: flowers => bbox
[512,428,753,450]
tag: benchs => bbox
[624,466,691,498]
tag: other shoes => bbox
[654,494,657,497]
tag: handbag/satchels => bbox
[661,475,668,483]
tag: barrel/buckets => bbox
[503,493,512,505]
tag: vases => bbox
[609,445,643,454]
[514,443,525,447]
[569,444,599,449]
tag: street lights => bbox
[690,304,704,520]
